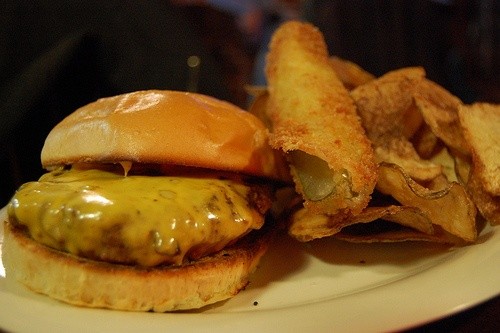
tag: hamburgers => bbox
[0,90,289,312]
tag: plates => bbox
[0,200,500,333]
